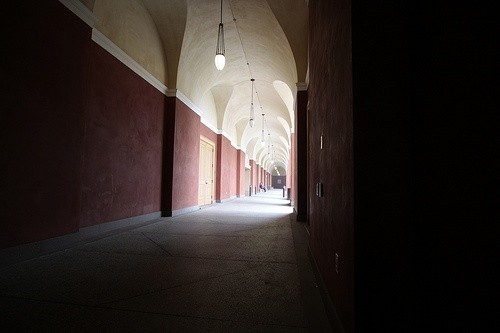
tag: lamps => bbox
[214,0,226,71]
[261,114,280,176]
[249,78,256,127]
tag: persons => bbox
[260,183,266,192]
